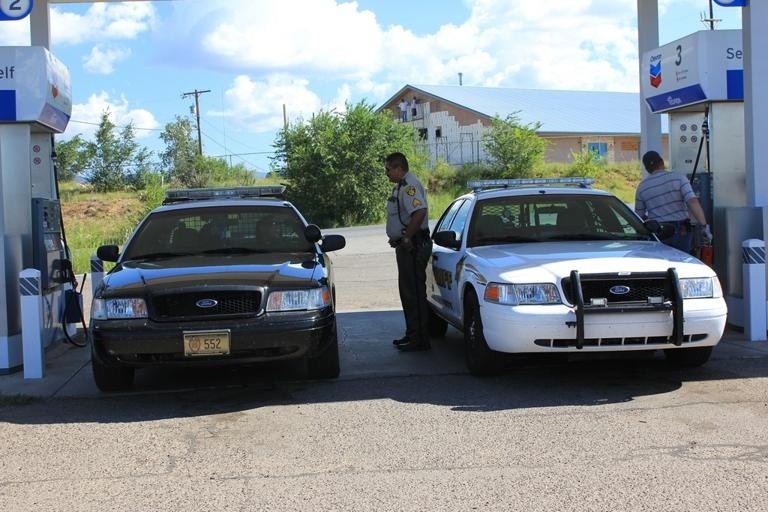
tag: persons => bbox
[634,150,714,254]
[384,151,435,350]
[239,215,301,250]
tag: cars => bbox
[423,174,729,378]
[87,183,348,396]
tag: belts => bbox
[390,238,402,247]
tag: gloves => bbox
[700,224,713,240]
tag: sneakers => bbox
[393,337,432,350]
